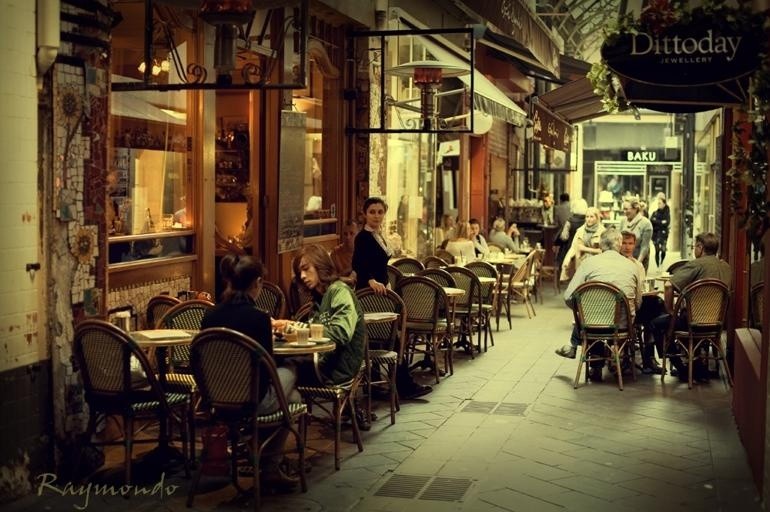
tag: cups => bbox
[455,248,509,266]
[297,329,310,344]
[644,280,651,292]
[311,324,324,338]
[536,243,540,249]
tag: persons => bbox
[202,253,306,491]
[391,176,735,383]
[331,219,360,288]
[351,197,432,399]
[294,242,367,383]
[172,192,188,222]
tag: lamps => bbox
[378,54,471,133]
[196,0,262,79]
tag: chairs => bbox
[72,321,190,498]
[188,327,306,509]
[561,252,733,397]
[144,239,541,471]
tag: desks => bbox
[130,330,335,448]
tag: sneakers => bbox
[588,367,602,380]
[364,381,391,401]
[399,383,434,401]
[670,367,711,383]
[554,344,577,359]
[642,358,668,374]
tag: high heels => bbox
[260,467,301,496]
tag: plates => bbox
[309,337,330,344]
[290,342,317,347]
[140,329,190,340]
